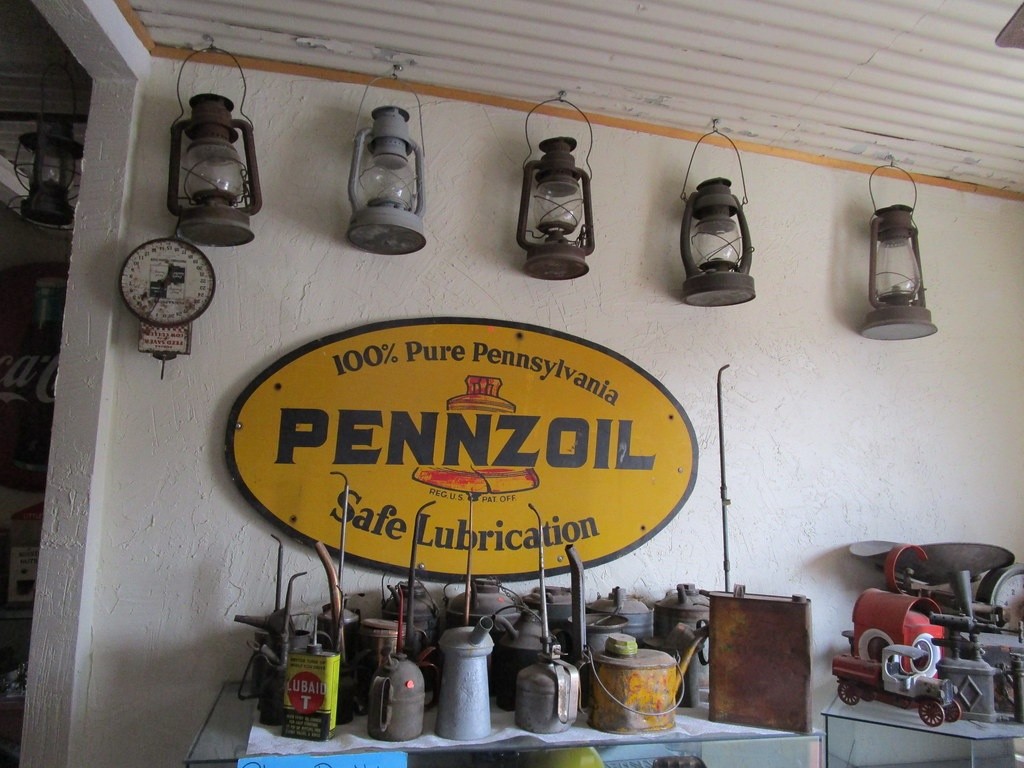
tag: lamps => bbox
[346,76,426,255]
[860,165,938,340]
[7,64,84,230]
[166,47,263,247]
[517,99,595,280]
[680,130,756,307]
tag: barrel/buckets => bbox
[707,583,814,735]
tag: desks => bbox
[183,680,828,768]
[821,685,1024,768]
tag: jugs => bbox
[575,628,706,734]
